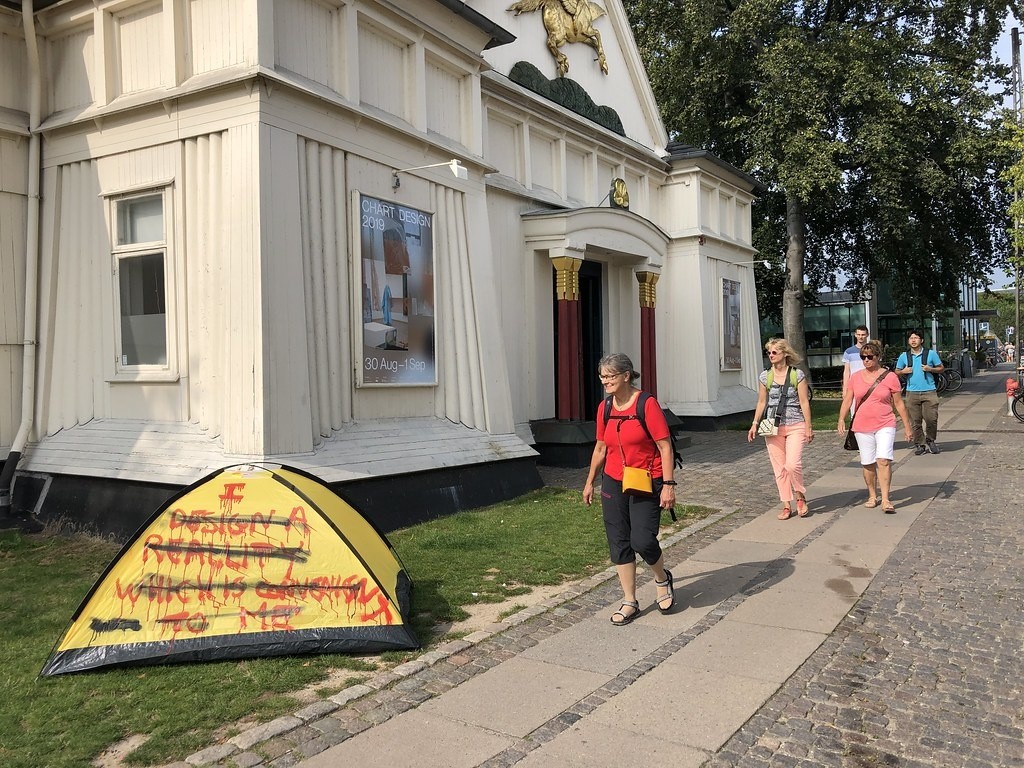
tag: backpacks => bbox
[604,391,683,470]
[906,348,939,386]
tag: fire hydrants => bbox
[1006,378,1020,416]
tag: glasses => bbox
[910,336,921,340]
[860,355,875,360]
[766,351,778,356]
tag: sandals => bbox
[778,508,792,519]
[865,497,878,508]
[797,498,809,516]
[654,568,674,612]
[609,600,641,625]
[881,499,894,513]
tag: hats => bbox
[1005,342,1008,345]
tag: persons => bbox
[977,346,996,367]
[582,353,677,627]
[999,342,1015,362]
[842,325,869,419]
[838,340,914,512]
[748,337,815,520]
[895,331,944,455]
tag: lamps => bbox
[729,260,771,269]
[392,158,469,179]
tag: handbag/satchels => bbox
[758,418,778,436]
[844,428,859,450]
[622,466,653,494]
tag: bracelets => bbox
[752,421,759,425]
[663,480,677,485]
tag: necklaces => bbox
[774,369,787,377]
[616,392,632,410]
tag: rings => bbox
[911,437,913,438]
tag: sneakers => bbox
[915,445,926,456]
[926,438,939,454]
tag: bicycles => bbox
[897,369,962,393]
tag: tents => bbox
[32,462,420,686]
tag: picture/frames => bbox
[351,187,441,390]
[720,276,743,371]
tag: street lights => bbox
[844,303,853,346]
